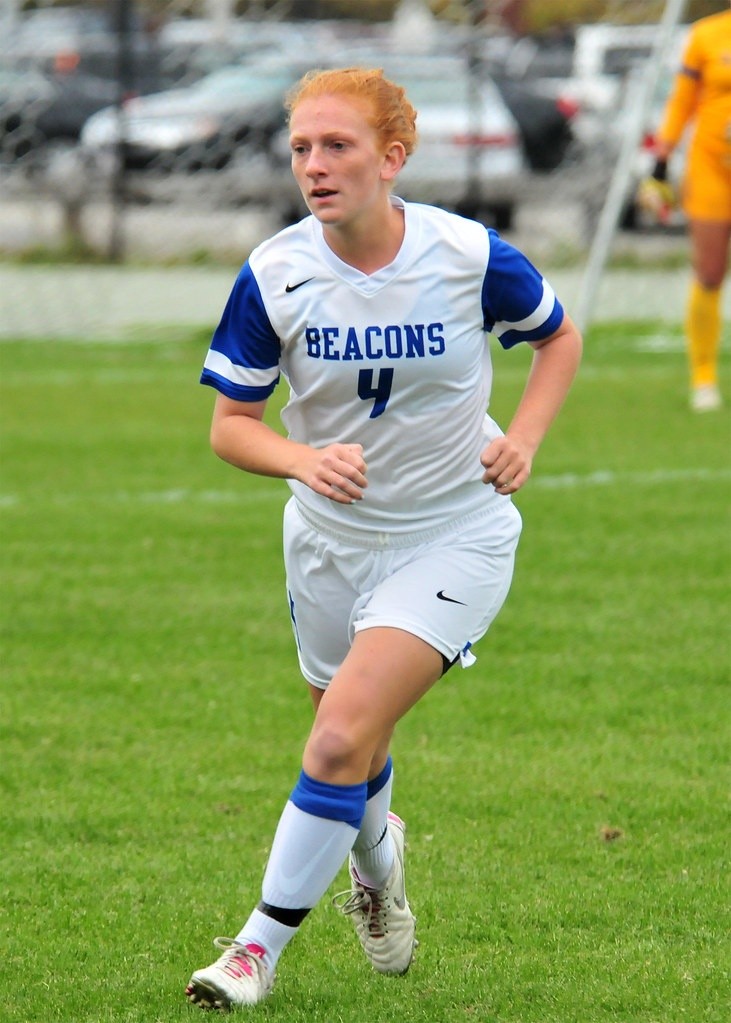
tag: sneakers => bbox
[184,939,275,1015]
[347,807,420,979]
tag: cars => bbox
[0,2,698,244]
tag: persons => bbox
[176,64,586,1016]
[641,8,731,413]
